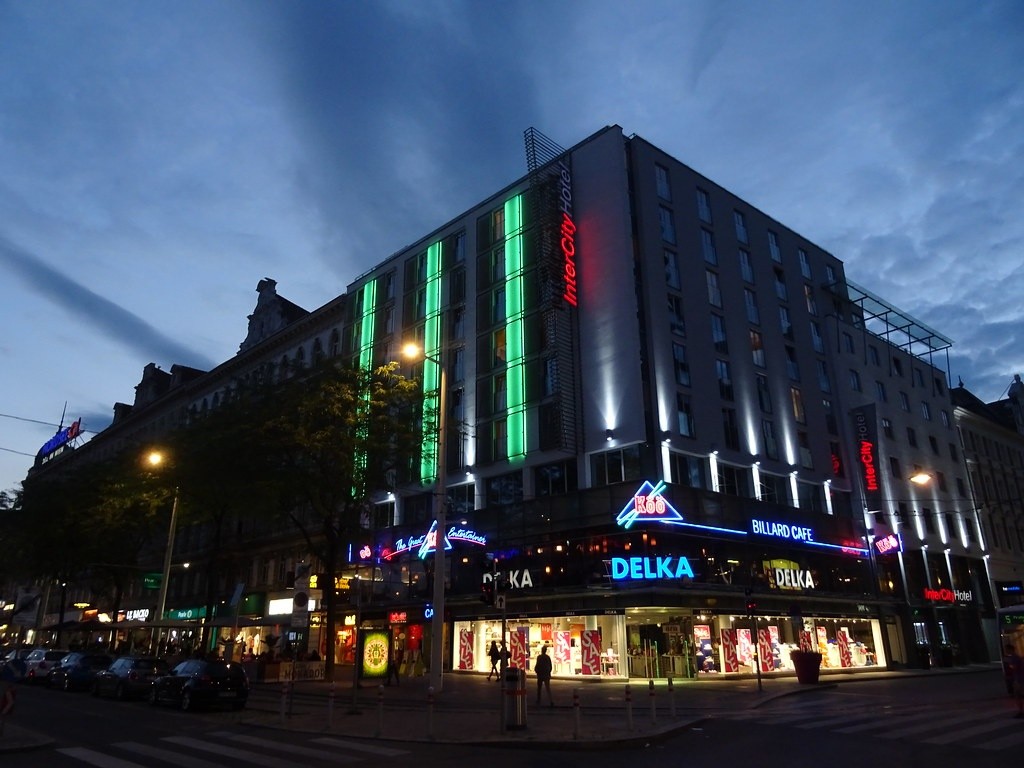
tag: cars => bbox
[0,647,251,711]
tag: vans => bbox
[997,605,1024,694]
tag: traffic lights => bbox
[746,599,759,616]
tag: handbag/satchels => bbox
[506,651,512,658]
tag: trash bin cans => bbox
[500,666,529,732]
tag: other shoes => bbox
[487,677,490,681]
[495,678,499,681]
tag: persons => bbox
[500,641,508,679]
[69,636,321,661]
[0,668,18,737]
[1004,644,1024,718]
[487,641,501,682]
[535,646,554,706]
[928,639,961,667]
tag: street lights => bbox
[387,332,443,694]
[140,444,182,653]
